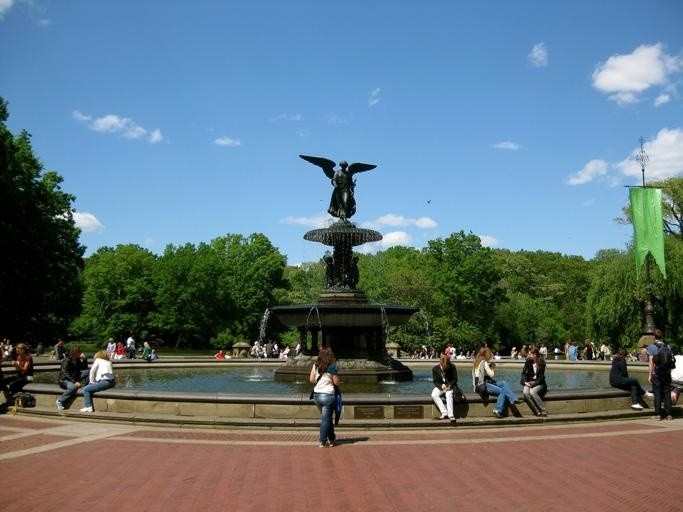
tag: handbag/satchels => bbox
[15,392,35,407]
[453,385,466,403]
[310,390,314,400]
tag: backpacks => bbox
[651,342,676,371]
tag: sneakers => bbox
[492,408,503,418]
[55,399,65,410]
[536,410,549,417]
[643,391,654,398]
[80,405,94,413]
[651,415,661,421]
[435,414,448,419]
[631,402,644,408]
[450,416,457,422]
[319,440,336,446]
[665,415,673,421]
[513,400,524,404]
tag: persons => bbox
[333,160,356,223]
[519,346,548,416]
[79,350,116,413]
[55,347,90,411]
[609,348,655,409]
[647,329,673,421]
[1,343,33,394]
[494,335,649,363]
[472,347,524,418]
[310,347,340,447]
[326,257,333,288]
[0,335,158,362]
[430,352,462,421]
[352,256,359,289]
[411,343,467,360]
[214,341,302,359]
